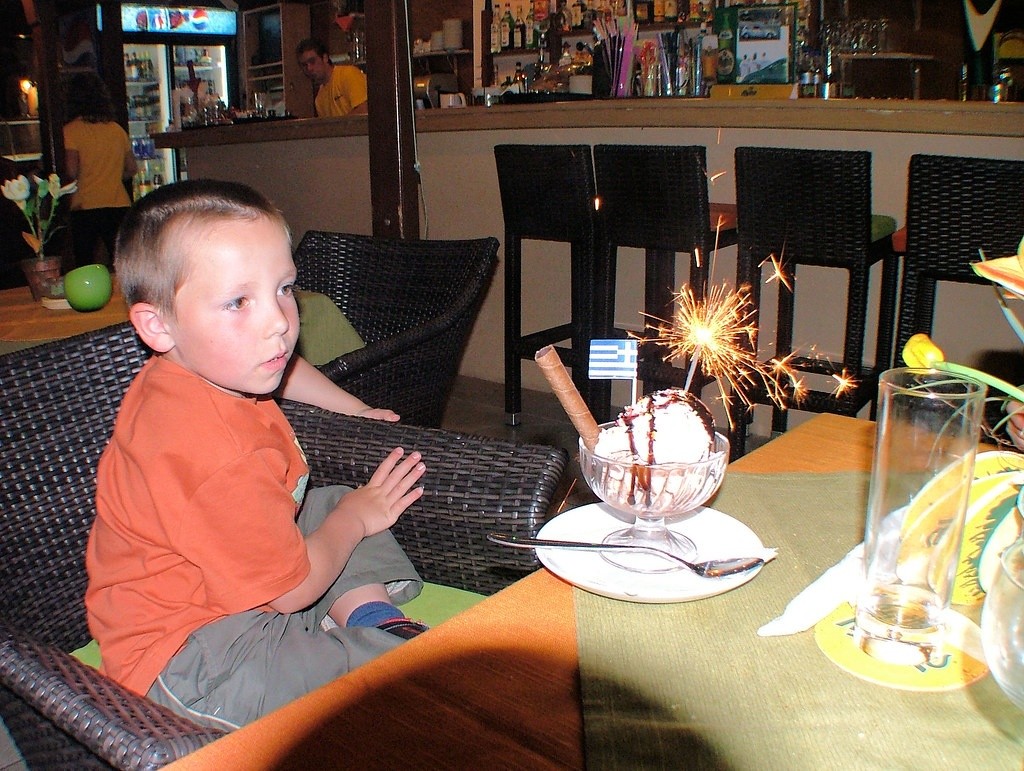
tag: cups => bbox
[854,367,988,642]
[981,542,1023,710]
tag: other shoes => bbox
[378,618,427,639]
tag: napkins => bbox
[754,505,906,638]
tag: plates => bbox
[535,503,764,603]
[432,20,463,51]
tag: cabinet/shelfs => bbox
[481,0,821,88]
[243,3,315,118]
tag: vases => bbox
[982,535,1022,711]
[22,256,61,303]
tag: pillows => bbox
[296,292,365,368]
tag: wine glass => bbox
[579,422,730,572]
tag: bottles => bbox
[490,4,501,53]
[501,76,511,87]
[526,0,534,48]
[123,51,155,82]
[795,25,809,73]
[559,42,572,65]
[717,14,733,49]
[126,82,161,120]
[568,0,719,29]
[130,122,162,202]
[813,51,824,97]
[800,47,814,97]
[818,17,888,53]
[514,6,526,49]
[824,64,836,99]
[513,61,525,93]
[501,3,514,50]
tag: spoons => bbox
[487,531,764,578]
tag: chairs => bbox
[0,319,569,771]
[293,229,499,430]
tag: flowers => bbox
[902,238,1023,467]
[1,174,78,260]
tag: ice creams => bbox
[534,344,727,515]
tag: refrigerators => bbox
[93,3,241,202]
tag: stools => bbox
[494,143,1023,466]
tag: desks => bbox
[151,410,1023,770]
[0,270,128,356]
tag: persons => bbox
[1005,384,1024,450]
[58,73,137,273]
[295,37,368,115]
[83,179,429,733]
